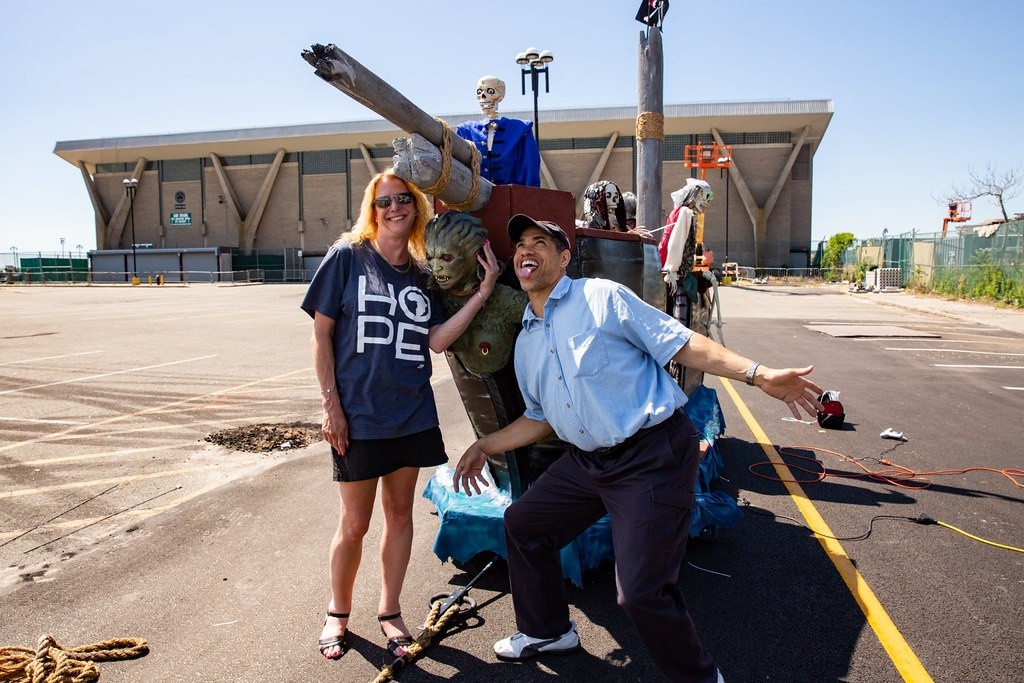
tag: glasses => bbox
[373,192,412,208]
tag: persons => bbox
[453,212,826,683]
[300,171,500,659]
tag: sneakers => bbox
[493,620,581,660]
[715,666,727,683]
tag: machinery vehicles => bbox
[941,201,972,238]
[684,142,739,287]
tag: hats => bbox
[507,214,571,250]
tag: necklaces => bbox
[373,238,412,274]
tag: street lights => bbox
[10,247,19,267]
[516,49,554,150]
[76,245,83,259]
[60,238,65,257]
[123,178,140,277]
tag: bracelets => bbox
[477,290,486,306]
[745,361,760,386]
[321,387,337,401]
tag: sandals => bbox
[319,612,349,659]
[378,612,414,658]
